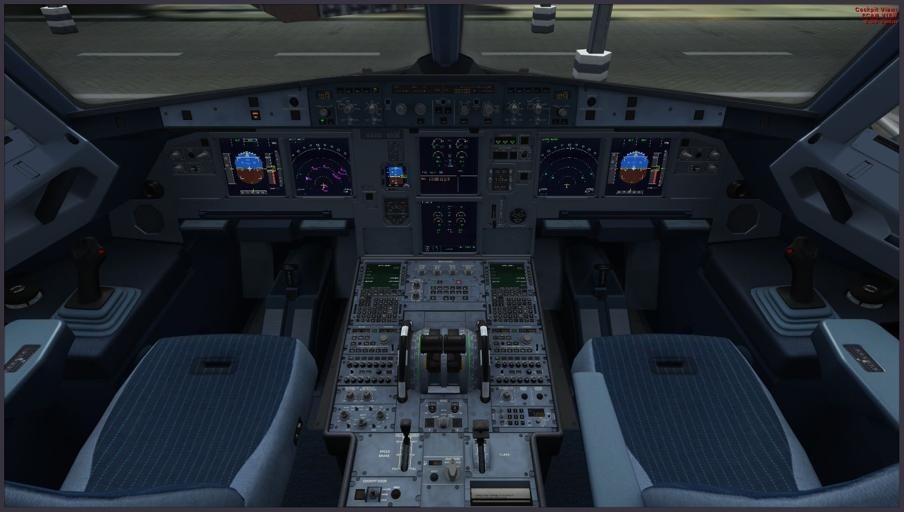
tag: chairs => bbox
[567,314,902,510]
[1,315,319,511]
